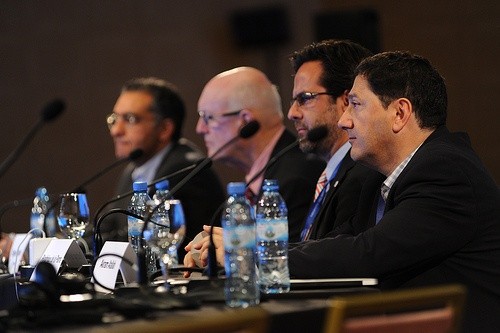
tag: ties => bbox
[303,171,327,243]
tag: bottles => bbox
[127,180,174,284]
[221,182,261,309]
[30,187,56,239]
[257,179,291,294]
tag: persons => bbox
[185,49,500,333]
[0,40,388,284]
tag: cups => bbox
[21,265,35,278]
[56,194,90,245]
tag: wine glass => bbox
[144,200,187,285]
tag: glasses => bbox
[290,90,341,107]
[107,112,163,125]
[196,108,249,125]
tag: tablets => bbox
[290,277,379,289]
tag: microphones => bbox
[0,99,65,177]
[41,119,330,299]
